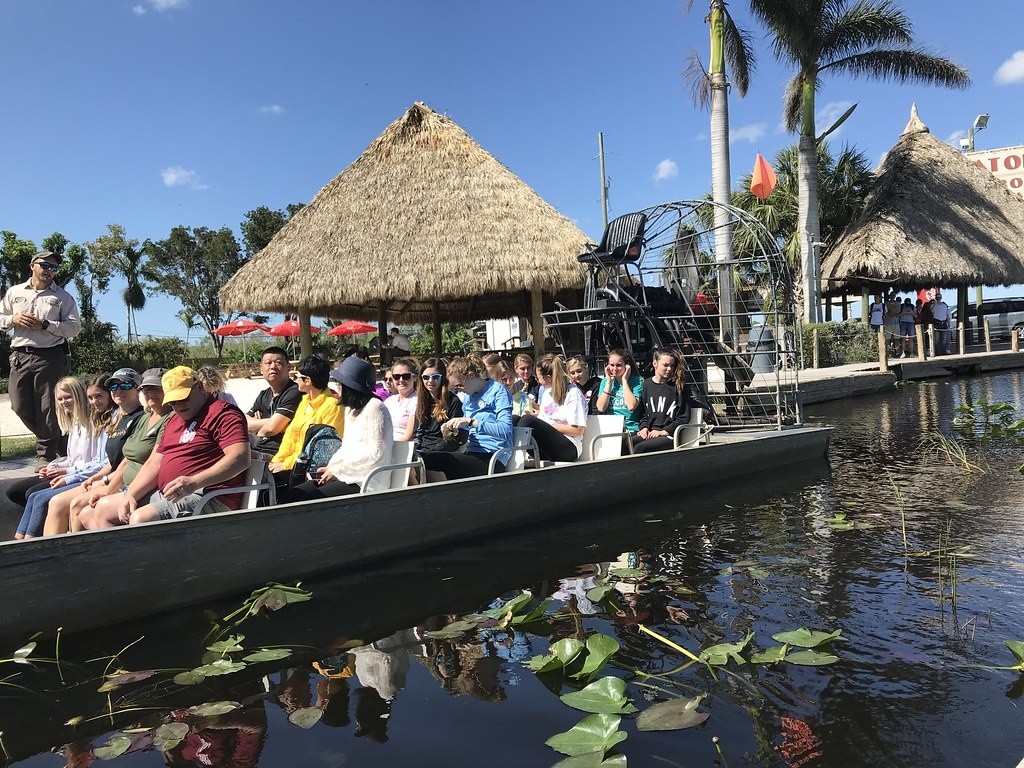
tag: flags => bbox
[750,153,777,200]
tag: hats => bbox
[104,368,141,388]
[160,365,199,405]
[328,357,376,393]
[137,367,168,389]
[32,250,63,265]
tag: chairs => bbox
[577,212,647,305]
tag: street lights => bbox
[967,112,992,346]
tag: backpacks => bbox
[290,422,343,489]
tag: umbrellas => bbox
[265,319,320,359]
[327,320,377,346]
[210,319,269,363]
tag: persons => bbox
[378,328,410,370]
[869,291,951,358]
[0,250,81,472]
[6,347,690,539]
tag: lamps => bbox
[973,114,990,135]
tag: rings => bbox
[176,491,179,496]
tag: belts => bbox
[15,346,35,353]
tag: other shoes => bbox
[899,353,905,358]
[380,366,389,371]
[910,354,918,358]
[35,454,54,473]
[946,349,951,354]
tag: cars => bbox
[947,297,1024,341]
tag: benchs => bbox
[150,405,708,518]
[218,363,378,378]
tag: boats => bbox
[0,458,834,764]
[0,196,836,650]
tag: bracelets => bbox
[469,418,475,426]
[603,391,611,395]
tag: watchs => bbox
[103,475,109,484]
[42,319,50,330]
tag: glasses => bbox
[168,384,195,406]
[107,382,134,391]
[32,261,59,272]
[451,374,468,392]
[936,296,942,299]
[391,332,394,334]
[294,373,309,381]
[421,373,444,381]
[382,377,393,382]
[566,354,582,364]
[392,372,417,381]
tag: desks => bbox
[222,354,380,378]
